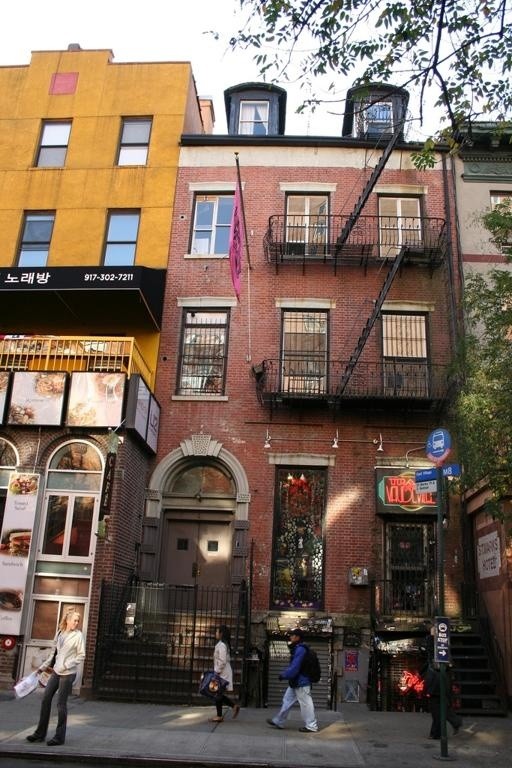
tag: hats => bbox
[286,629,305,636]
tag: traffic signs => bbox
[436,617,449,656]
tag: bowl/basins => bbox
[0,589,22,611]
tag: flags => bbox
[230,176,245,303]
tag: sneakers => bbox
[208,716,224,722]
[26,734,61,746]
[231,704,240,719]
[266,718,283,730]
[298,727,314,733]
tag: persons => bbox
[265,628,321,732]
[413,618,463,741]
[199,624,239,723]
[26,607,86,745]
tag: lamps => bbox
[263,426,386,452]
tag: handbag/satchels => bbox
[199,671,230,703]
[38,665,54,688]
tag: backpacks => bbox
[295,645,322,684]
